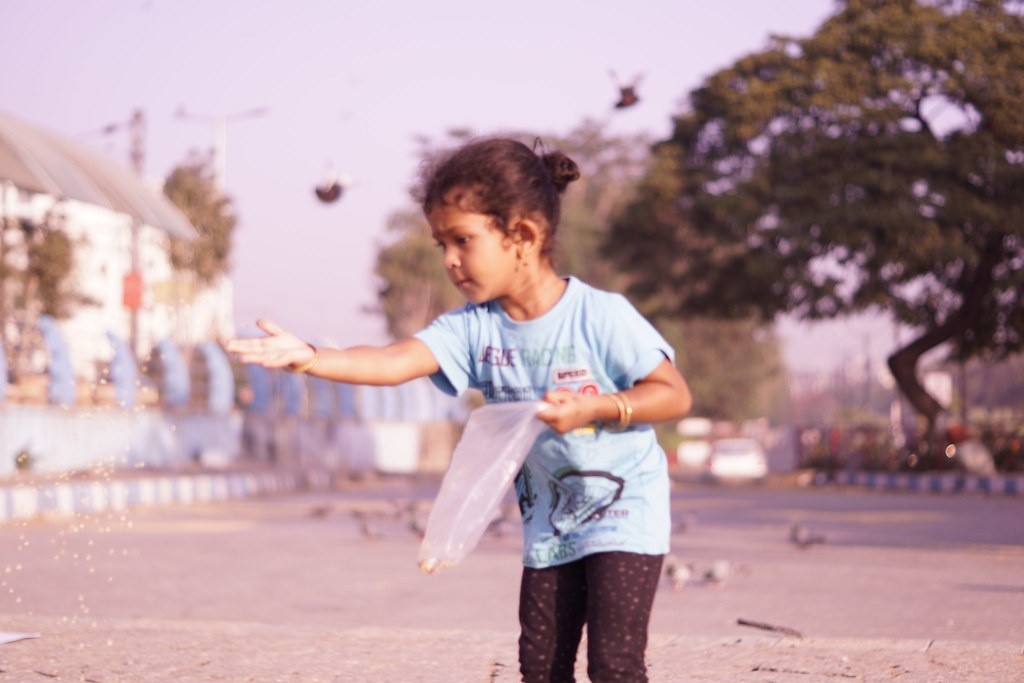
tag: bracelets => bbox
[609,392,632,433]
[285,338,318,374]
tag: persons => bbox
[226,135,693,683]
